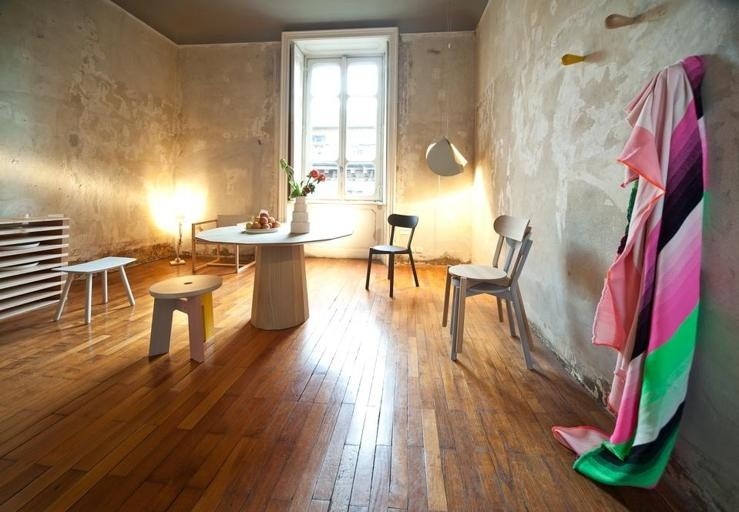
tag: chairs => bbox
[190,213,267,275]
[364,211,421,299]
[439,213,539,372]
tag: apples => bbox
[246,211,280,229]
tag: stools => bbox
[146,273,223,364]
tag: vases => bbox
[289,195,311,235]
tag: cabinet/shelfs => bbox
[0,212,72,324]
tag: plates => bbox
[0,238,41,274]
[233,222,280,234]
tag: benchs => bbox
[50,255,139,326]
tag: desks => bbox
[194,221,353,332]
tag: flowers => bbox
[279,157,328,200]
